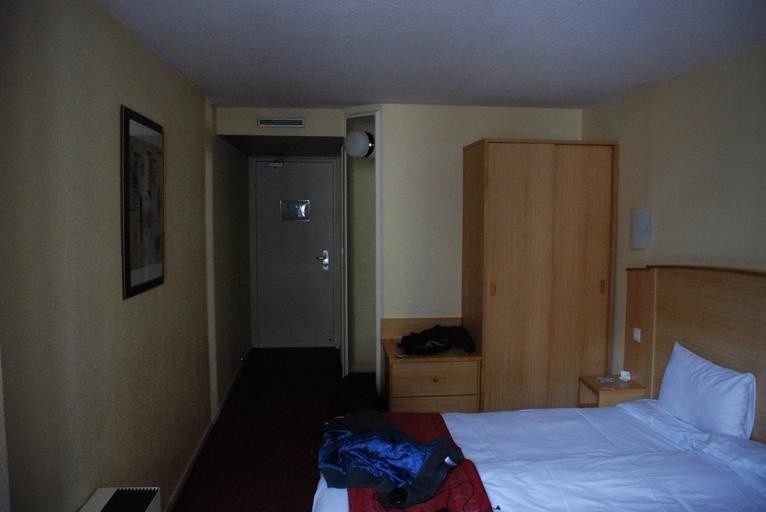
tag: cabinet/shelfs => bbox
[463,140,619,411]
[381,316,479,414]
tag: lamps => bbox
[345,128,374,162]
[628,208,651,250]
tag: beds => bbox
[308,263,765,511]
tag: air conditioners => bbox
[77,486,162,512]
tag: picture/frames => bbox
[117,102,168,301]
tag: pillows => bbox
[658,341,756,437]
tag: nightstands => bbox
[576,370,641,407]
